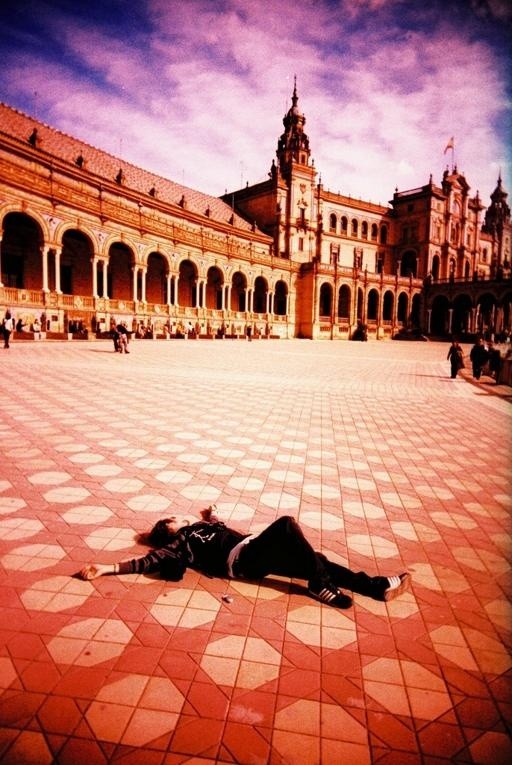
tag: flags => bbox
[443,137,453,153]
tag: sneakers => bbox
[370,572,411,601]
[307,583,354,609]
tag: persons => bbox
[0,312,13,349]
[446,335,512,385]
[77,505,413,610]
[0,314,274,355]
